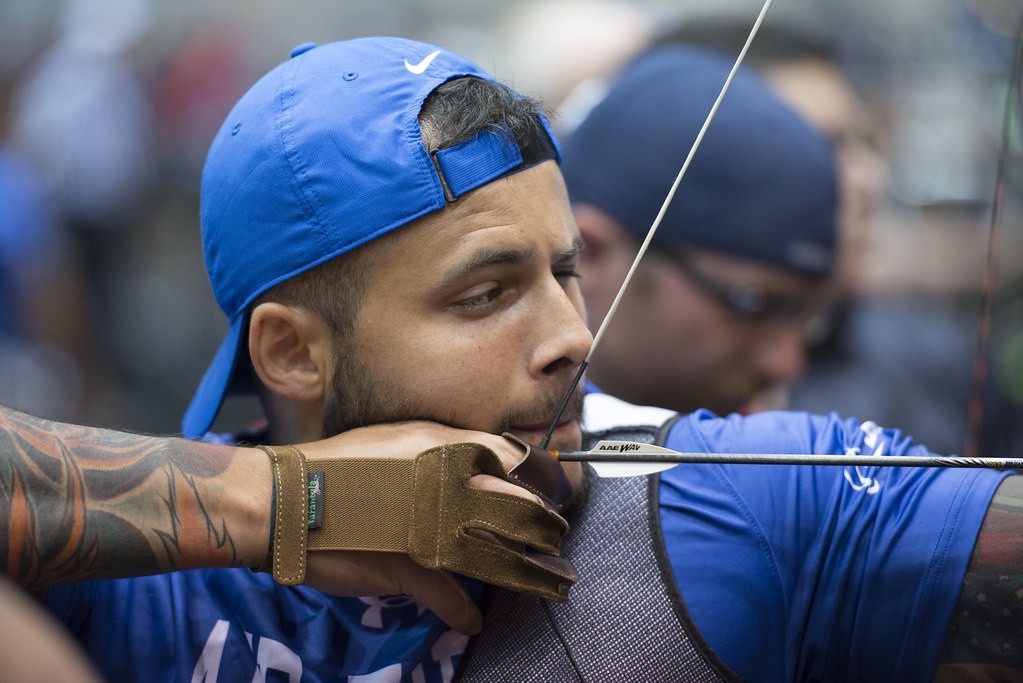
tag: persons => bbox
[555,23,1023,682]
[0,37,1023,682]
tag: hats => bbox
[178,36,554,440]
[558,41,836,274]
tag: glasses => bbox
[660,244,835,344]
